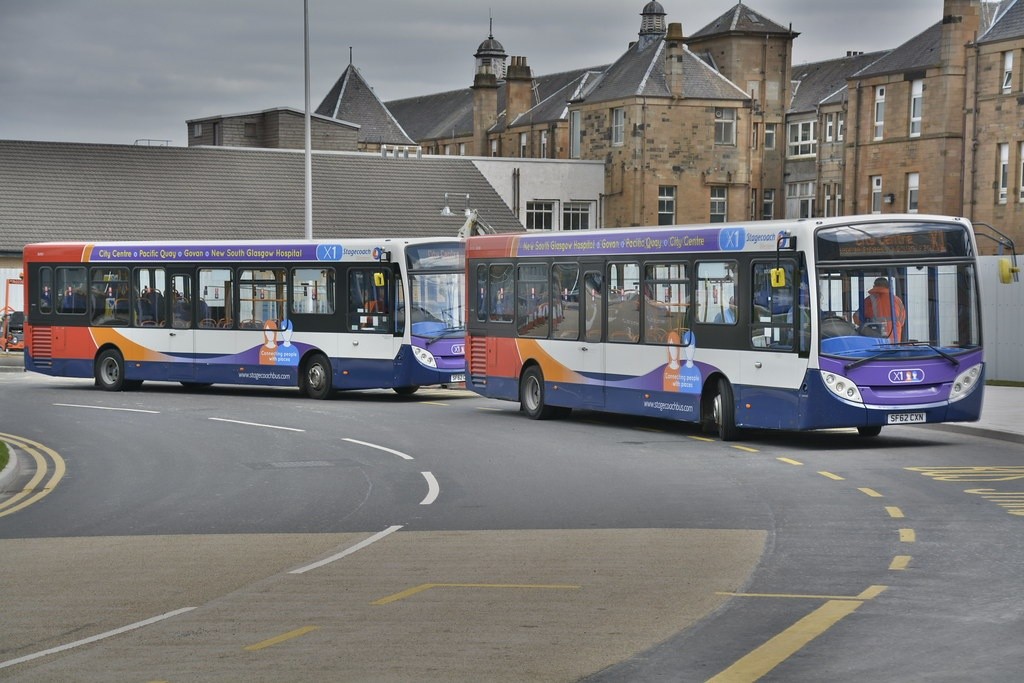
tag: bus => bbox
[460,212,1021,443]
[18,232,465,402]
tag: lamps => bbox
[883,192,895,205]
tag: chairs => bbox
[44,284,280,329]
[516,284,685,342]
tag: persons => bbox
[714,296,739,325]
[852,277,904,349]
